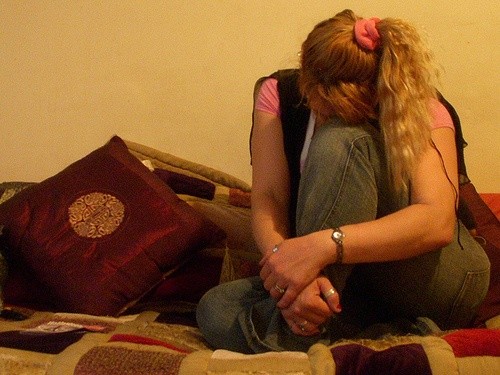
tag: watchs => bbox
[330,222,345,267]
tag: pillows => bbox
[0,135,229,317]
[423,184,500,328]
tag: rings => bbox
[273,285,285,294]
[324,288,340,298]
[270,245,280,253]
[298,321,310,327]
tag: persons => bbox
[197,7,491,356]
